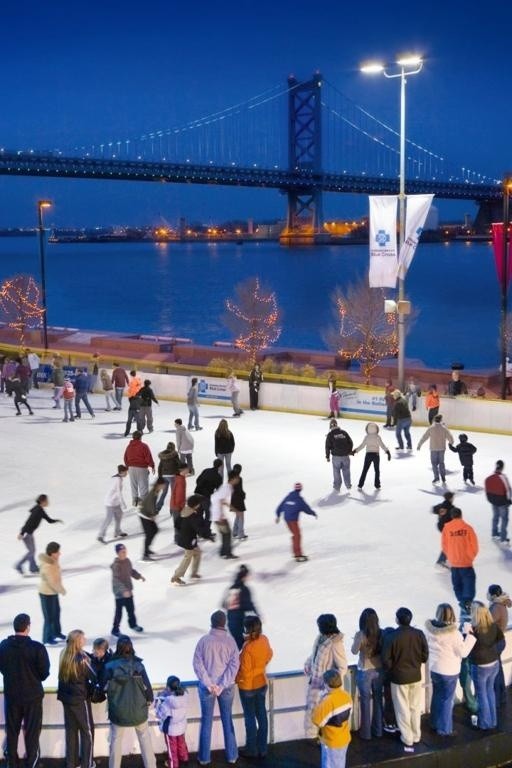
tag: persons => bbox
[1,347,41,416]
[50,352,95,422]
[308,668,354,768]
[89,638,114,693]
[13,494,63,574]
[237,616,273,758]
[485,460,511,542]
[193,609,241,765]
[249,362,264,409]
[431,490,481,612]
[102,634,157,767]
[97,418,248,585]
[100,360,160,437]
[223,565,260,649]
[154,674,191,767]
[39,541,66,644]
[276,482,317,563]
[111,544,146,636]
[351,605,383,739]
[327,371,343,419]
[56,626,100,767]
[301,612,347,744]
[0,612,51,767]
[224,367,244,416]
[426,582,511,735]
[325,419,392,489]
[380,605,430,746]
[384,378,476,484]
[187,378,202,431]
[444,372,468,396]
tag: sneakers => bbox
[124,430,154,437]
[333,485,353,492]
[110,631,124,639]
[393,735,422,746]
[188,425,203,431]
[142,550,156,563]
[165,761,178,768]
[53,634,68,642]
[232,406,259,416]
[357,486,381,491]
[98,536,109,545]
[476,723,498,730]
[490,534,510,547]
[113,532,128,538]
[293,555,308,563]
[396,446,412,450]
[328,415,341,418]
[190,573,201,580]
[229,759,238,763]
[463,477,474,483]
[130,624,143,632]
[53,405,96,422]
[384,726,399,733]
[171,576,187,586]
[15,566,40,576]
[105,407,121,411]
[358,731,384,741]
[219,533,248,560]
[199,760,211,764]
[43,640,59,647]
[432,478,446,483]
[430,726,459,737]
[239,748,269,758]
[0,387,34,415]
[383,424,397,427]
[132,500,146,508]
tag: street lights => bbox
[500,176,512,402]
[37,198,53,350]
[357,50,426,390]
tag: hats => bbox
[444,492,455,499]
[496,461,504,469]
[390,389,402,400]
[115,544,126,551]
[459,433,468,442]
[294,482,302,491]
[451,508,461,515]
[435,415,442,422]
[330,419,338,429]
